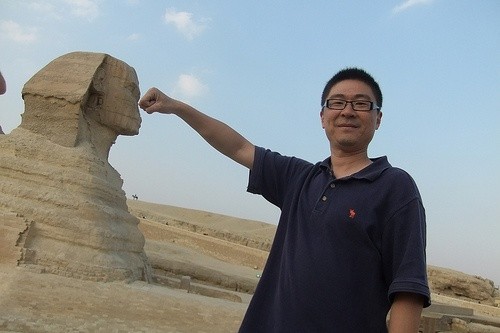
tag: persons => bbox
[139,68,431,333]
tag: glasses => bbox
[322,99,379,111]
